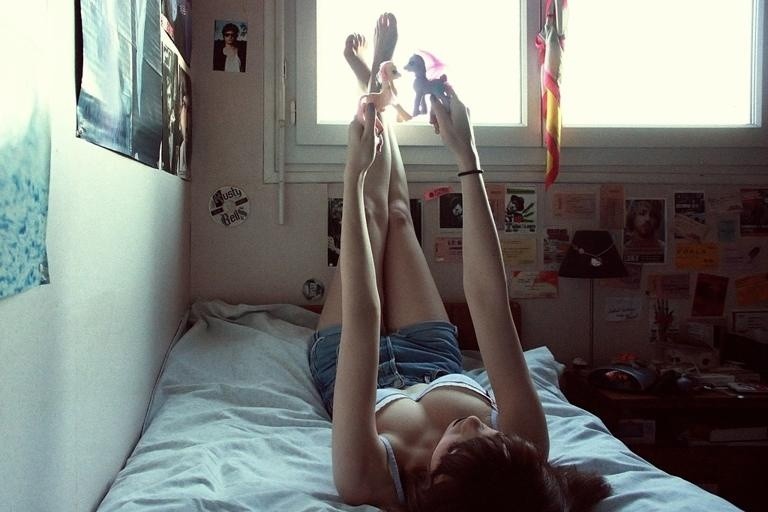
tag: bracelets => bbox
[458,169,483,177]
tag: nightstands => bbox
[579,358,768,512]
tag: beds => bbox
[98,301,737,511]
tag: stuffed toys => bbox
[358,54,451,132]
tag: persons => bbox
[215,24,246,72]
[307,12,570,511]
[166,81,189,178]
[327,201,342,254]
[625,200,665,256]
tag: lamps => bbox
[558,231,628,387]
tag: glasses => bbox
[224,32,237,37]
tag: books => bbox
[694,366,768,396]
[709,426,766,442]
